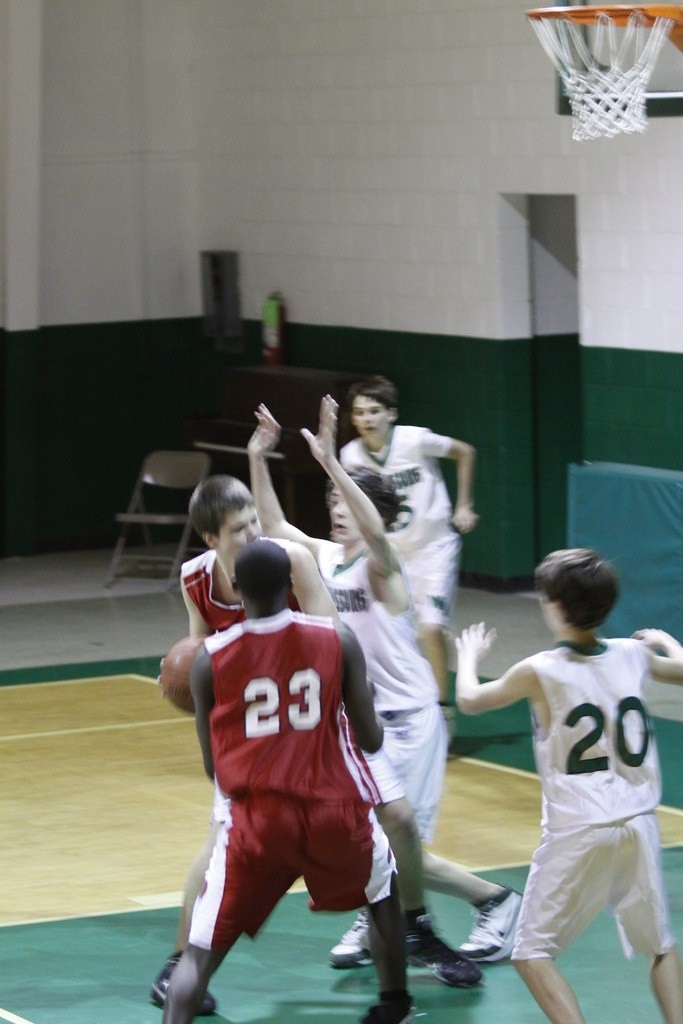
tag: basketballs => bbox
[162,635,208,713]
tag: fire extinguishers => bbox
[263,291,286,367]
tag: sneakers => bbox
[150,956,216,1014]
[358,985,413,1024]
[406,913,483,986]
[328,911,374,968]
[458,884,523,962]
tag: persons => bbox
[340,375,478,762]
[455,548,683,1024]
[162,539,415,1024]
[150,474,483,1016]
[248,394,524,965]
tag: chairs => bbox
[106,448,219,585]
